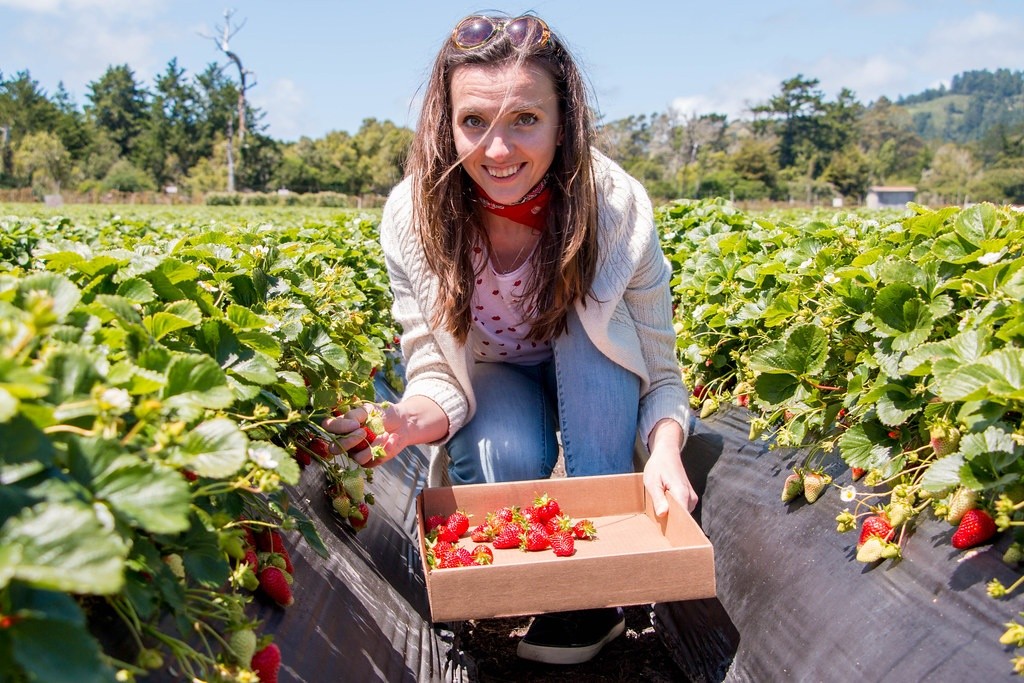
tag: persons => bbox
[322,14,704,666]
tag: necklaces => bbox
[479,210,537,275]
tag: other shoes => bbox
[519,607,625,664]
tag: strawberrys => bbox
[424,491,598,570]
[688,385,995,562]
[142,334,400,683]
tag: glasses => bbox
[451,13,551,54]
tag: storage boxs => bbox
[415,472,717,623]
